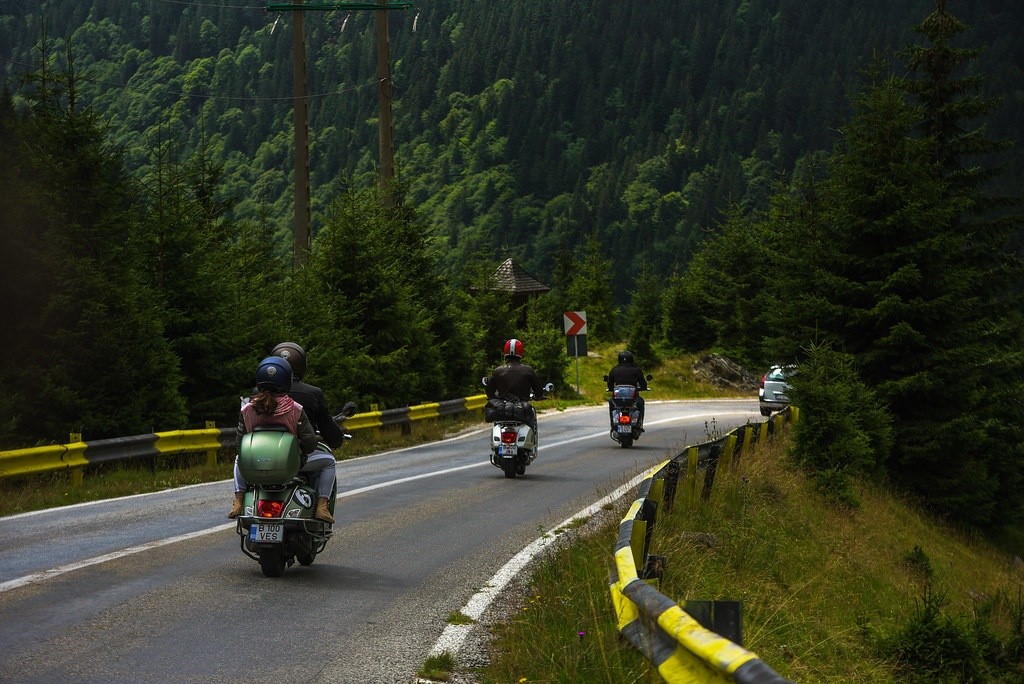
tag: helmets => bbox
[272,342,307,382]
[255,356,293,395]
[503,340,525,361]
[618,351,633,366]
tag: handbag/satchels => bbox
[484,398,533,423]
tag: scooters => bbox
[603,375,652,448]
[482,377,554,479]
[235,401,359,577]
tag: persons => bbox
[607,351,647,433]
[228,356,336,524]
[254,343,344,458]
[485,339,544,402]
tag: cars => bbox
[758,364,798,416]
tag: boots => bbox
[315,498,336,524]
[228,493,245,518]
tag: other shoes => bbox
[325,527,334,537]
[639,426,645,433]
